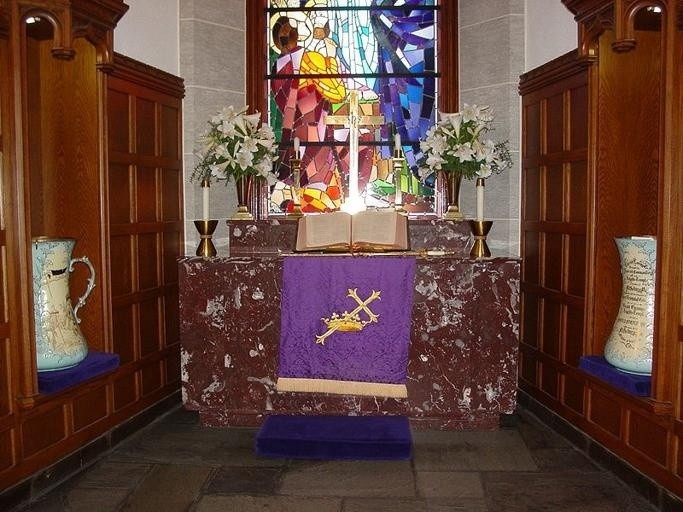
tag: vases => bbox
[445,173,462,221]
[232,174,253,219]
[604,228,657,377]
[24,228,100,374]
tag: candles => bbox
[201,186,209,219]
[477,186,484,220]
[394,134,400,149]
[293,137,299,150]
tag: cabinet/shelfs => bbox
[0,1,186,491]
[516,0,683,508]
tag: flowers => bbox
[415,103,514,182]
[189,106,278,186]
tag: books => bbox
[295,212,407,250]
